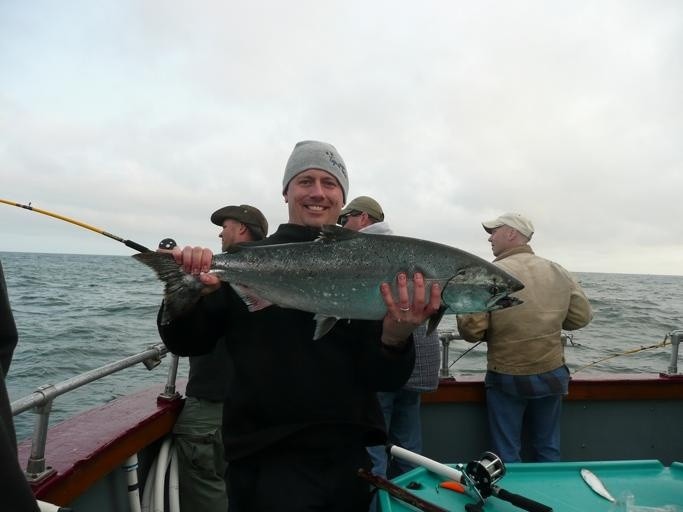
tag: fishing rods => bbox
[1,197,156,253]
[571,343,671,380]
[384,442,552,512]
[448,340,483,367]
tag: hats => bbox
[481,210,535,241]
[211,205,268,240]
[283,140,349,206]
[336,196,384,224]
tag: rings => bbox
[398,305,410,312]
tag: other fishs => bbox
[131,223,525,341]
[580,468,620,506]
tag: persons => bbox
[155,140,442,511]
[455,212,595,464]
[151,203,270,511]
[334,195,443,487]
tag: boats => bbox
[6,330,682,509]
